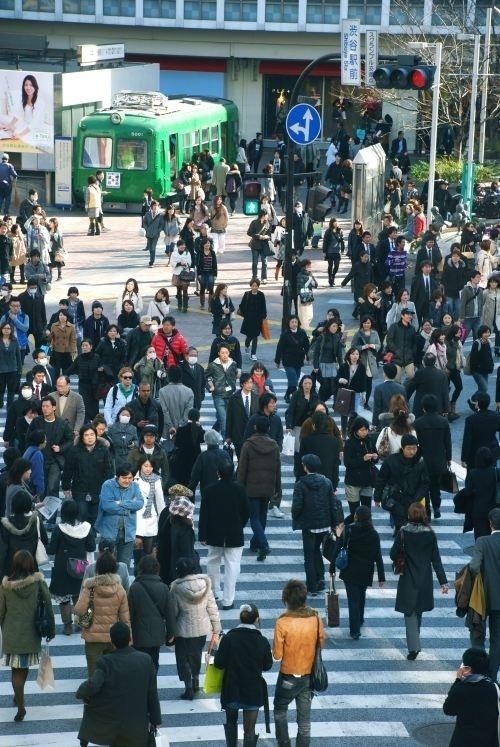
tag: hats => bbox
[301,454,322,473]
[142,424,158,436]
[204,429,223,445]
[167,483,194,498]
[169,496,196,517]
[401,308,415,315]
[19,382,35,392]
[92,301,103,312]
[140,315,154,326]
[440,179,450,185]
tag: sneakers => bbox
[245,346,257,361]
[284,394,290,404]
[63,621,73,636]
[433,505,441,518]
[258,549,270,561]
[467,398,478,412]
[149,262,154,268]
[267,506,285,518]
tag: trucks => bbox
[71,87,243,215]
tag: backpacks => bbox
[226,177,236,193]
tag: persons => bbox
[49,277,343,746]
[312,261,500,660]
[2,75,42,139]
[84,169,111,235]
[442,508,499,746]
[3,152,63,724]
[142,88,500,319]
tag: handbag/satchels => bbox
[211,185,217,195]
[261,319,270,340]
[272,218,280,227]
[237,308,245,320]
[383,338,394,364]
[36,539,49,567]
[312,649,328,692]
[325,592,340,626]
[381,483,401,510]
[454,321,465,343]
[494,482,500,504]
[245,164,251,172]
[392,552,406,575]
[311,370,323,394]
[322,536,336,561]
[384,201,391,213]
[184,185,192,195]
[463,351,473,375]
[307,349,323,362]
[375,353,385,368]
[300,291,314,303]
[263,241,275,256]
[203,664,225,694]
[179,271,196,282]
[334,388,355,417]
[16,216,28,234]
[378,436,391,459]
[36,602,51,637]
[282,434,295,456]
[341,182,352,193]
[175,354,186,366]
[198,188,206,201]
[42,345,54,355]
[55,251,68,262]
[138,227,146,237]
[66,557,89,579]
[92,375,115,400]
[313,222,323,237]
[334,547,350,569]
[229,311,236,321]
[172,178,184,190]
[441,469,459,493]
[206,219,212,227]
[461,251,474,259]
[36,652,56,693]
[74,606,94,629]
[370,465,380,487]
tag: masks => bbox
[38,358,47,366]
[29,288,37,294]
[188,357,198,365]
[120,415,131,425]
[149,352,157,360]
[459,663,472,679]
[21,389,33,398]
[1,291,8,296]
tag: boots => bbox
[340,198,348,214]
[181,685,200,700]
[243,733,259,747]
[448,401,460,423]
[329,267,338,287]
[182,294,189,313]
[200,293,205,310]
[223,724,238,747]
[274,265,280,280]
[208,293,214,312]
[87,223,95,236]
[175,295,182,311]
[337,197,344,211]
[132,548,144,577]
[95,222,100,235]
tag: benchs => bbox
[0,33,50,54]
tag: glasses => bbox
[121,375,133,380]
[3,327,12,330]
[10,304,21,308]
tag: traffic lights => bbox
[370,62,432,93]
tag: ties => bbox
[391,242,394,248]
[428,250,432,258]
[425,278,429,298]
[245,396,250,418]
[366,244,369,255]
[36,385,39,399]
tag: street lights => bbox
[453,29,479,226]
[403,38,445,238]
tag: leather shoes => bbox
[408,649,419,661]
